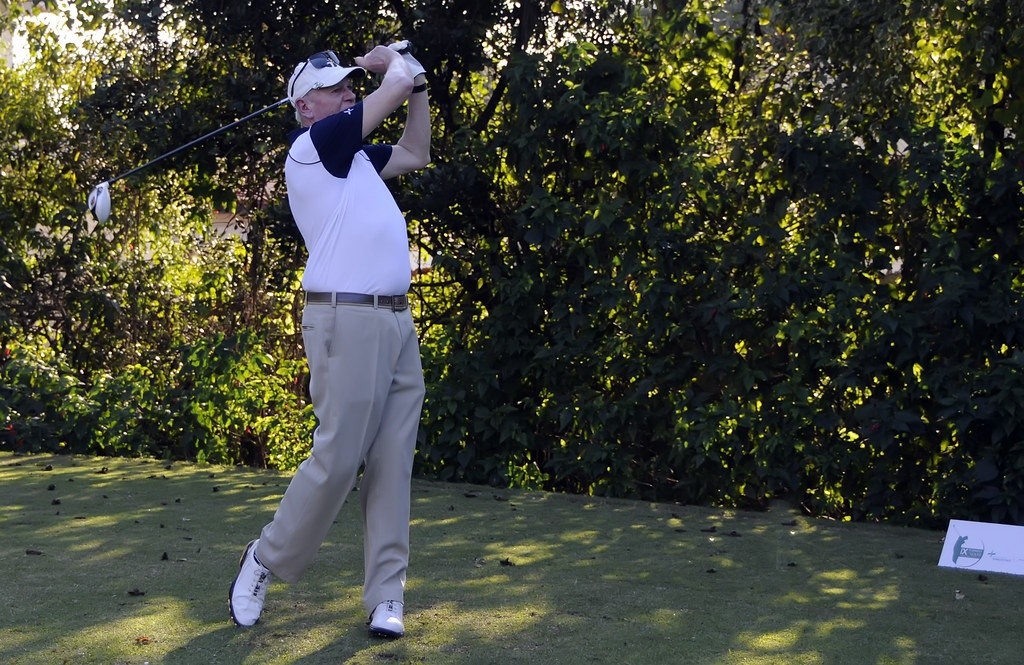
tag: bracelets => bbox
[412,82,427,94]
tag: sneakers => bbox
[370,600,405,636]
[228,539,272,628]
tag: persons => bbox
[228,40,431,638]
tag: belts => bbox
[304,292,408,312]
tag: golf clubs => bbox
[83,37,415,225]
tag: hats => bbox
[287,51,366,108]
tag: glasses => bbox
[291,50,340,97]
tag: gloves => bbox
[387,40,426,78]
